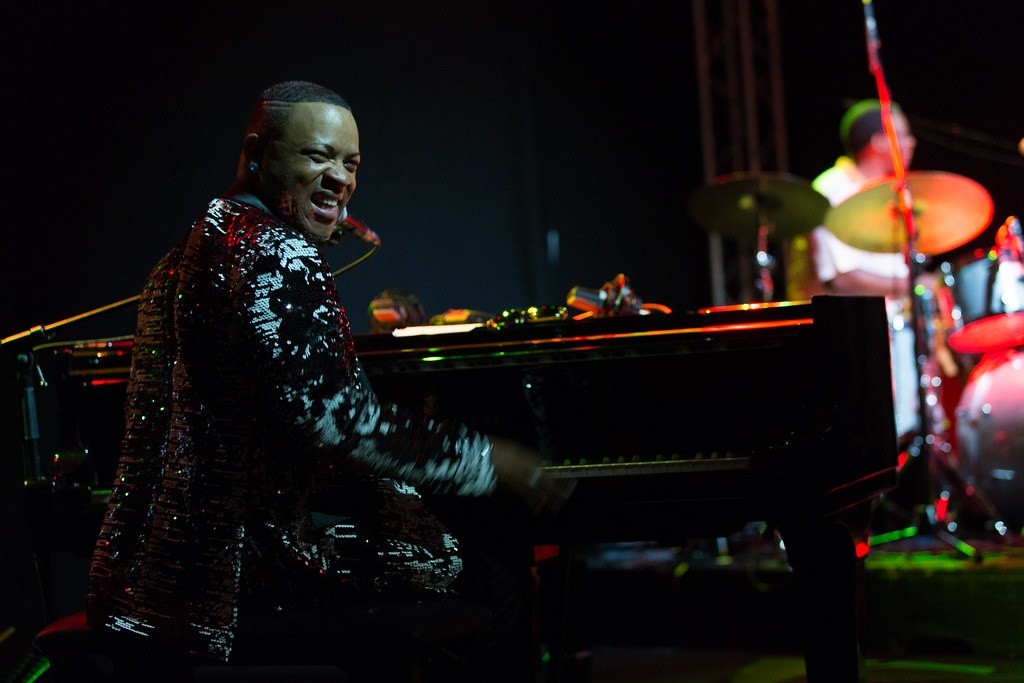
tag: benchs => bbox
[32,609,427,683]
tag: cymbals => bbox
[682,169,994,254]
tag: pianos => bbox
[11,288,902,683]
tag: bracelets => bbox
[892,277,900,298]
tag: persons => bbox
[811,100,967,539]
[86,80,575,683]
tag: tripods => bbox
[862,0,1014,563]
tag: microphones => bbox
[567,286,650,316]
[334,207,380,246]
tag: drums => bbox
[888,252,1024,532]
